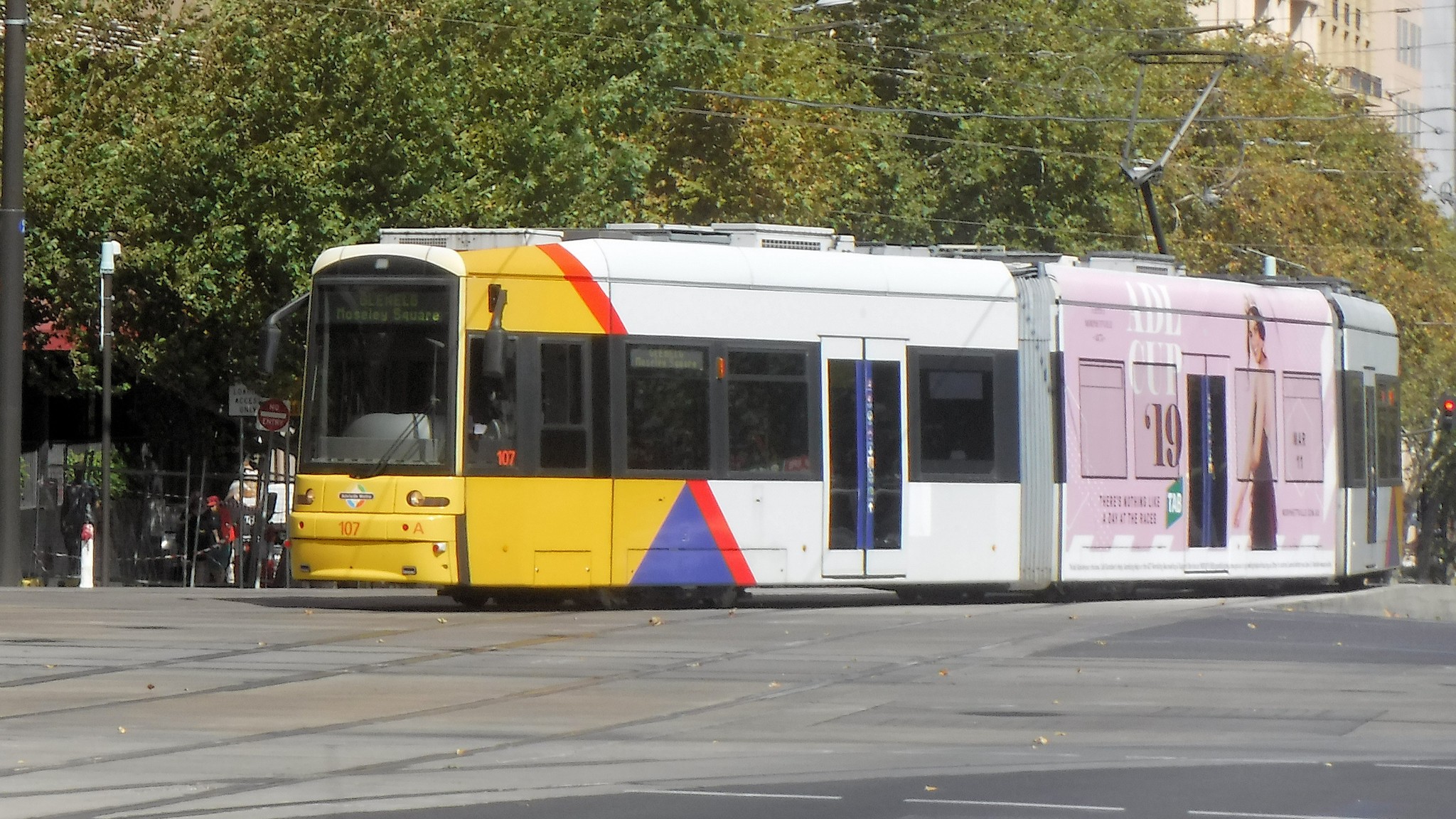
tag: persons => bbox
[178,491,275,588]
[1233,305,1279,550]
[61,482,102,579]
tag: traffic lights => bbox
[1438,393,1456,434]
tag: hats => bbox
[206,495,218,506]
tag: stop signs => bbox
[256,398,291,432]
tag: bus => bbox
[264,222,1403,611]
